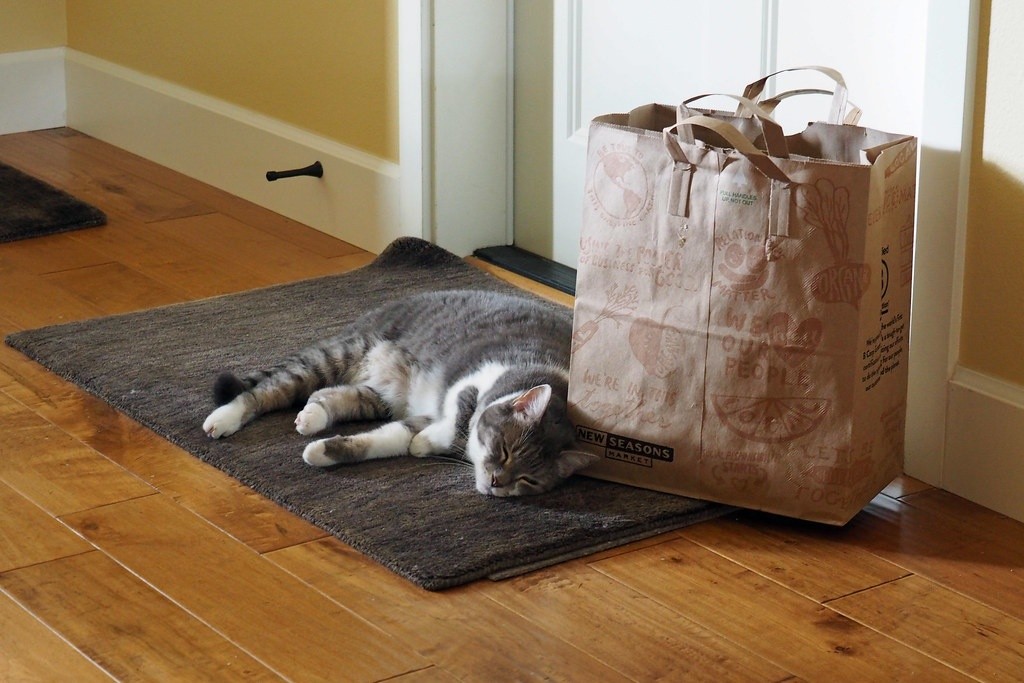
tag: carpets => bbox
[4,235,721,591]
[0,162,107,242]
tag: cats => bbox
[202,289,602,500]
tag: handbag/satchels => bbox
[565,64,917,527]
